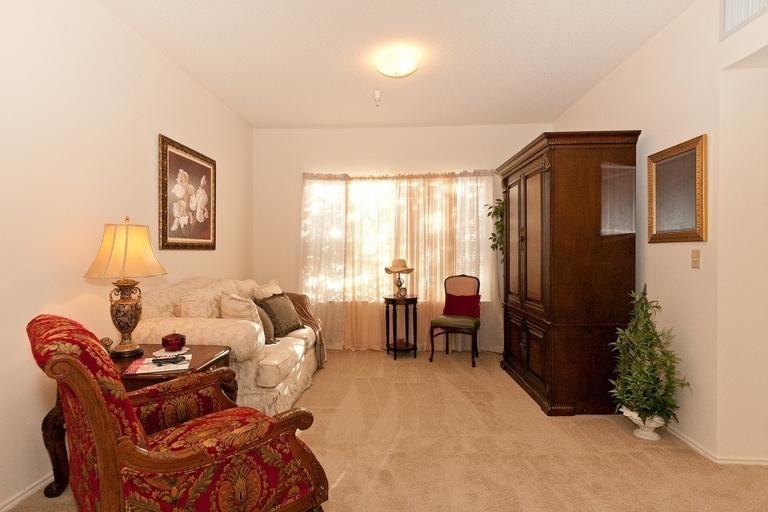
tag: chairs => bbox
[26,313,330,512]
[428,274,481,367]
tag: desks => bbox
[385,297,418,360]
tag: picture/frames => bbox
[159,134,217,251]
[647,134,709,243]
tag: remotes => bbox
[152,355,186,366]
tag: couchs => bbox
[131,277,326,417]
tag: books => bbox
[123,351,192,376]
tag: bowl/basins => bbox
[162,334,186,352]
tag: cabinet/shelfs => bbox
[495,129,642,417]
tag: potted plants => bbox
[607,282,694,441]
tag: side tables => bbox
[42,344,231,498]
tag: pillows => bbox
[220,280,303,345]
[443,293,481,317]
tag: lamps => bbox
[83,215,167,359]
[384,258,415,299]
